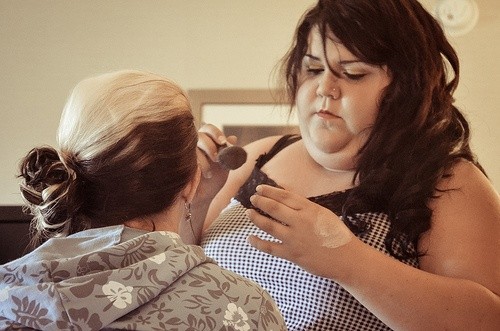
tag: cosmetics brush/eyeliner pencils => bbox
[199,121,248,171]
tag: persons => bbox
[177,0,500,331]
[0,69,290,331]
